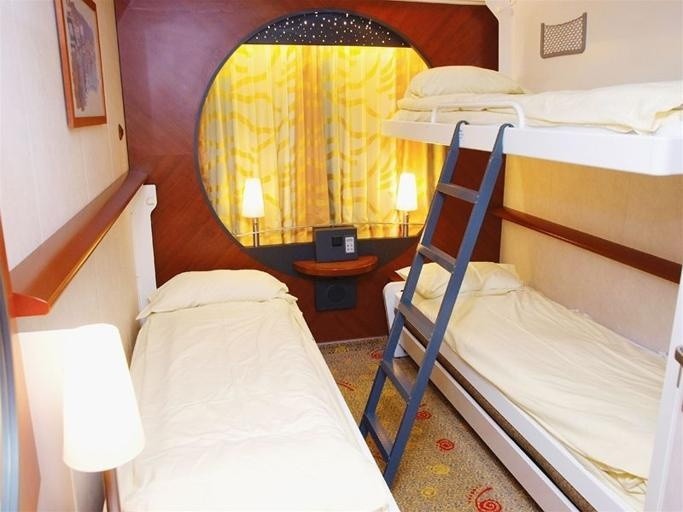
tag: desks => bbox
[293,256,378,277]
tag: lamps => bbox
[396,173,418,238]
[62,323,144,512]
[242,178,265,246]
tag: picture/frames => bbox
[54,0,107,128]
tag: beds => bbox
[382,81,683,512]
[105,298,388,512]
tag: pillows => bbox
[136,270,299,323]
[406,65,531,97]
[394,262,523,299]
